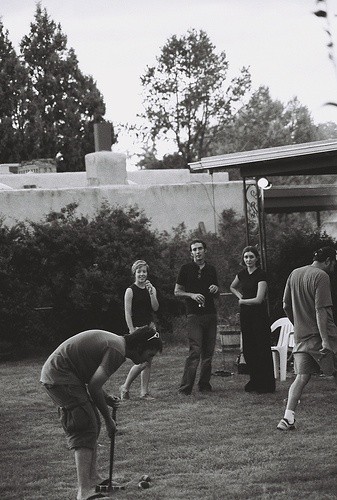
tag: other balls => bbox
[138,475,151,482]
[139,482,153,489]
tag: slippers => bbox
[86,493,115,500]
[97,478,120,487]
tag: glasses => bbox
[147,332,160,341]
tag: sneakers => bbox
[276,418,297,431]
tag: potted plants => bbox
[219,312,240,348]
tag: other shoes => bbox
[140,393,156,401]
[119,386,129,401]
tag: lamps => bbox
[256,175,272,190]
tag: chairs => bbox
[271,318,296,381]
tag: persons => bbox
[229,246,276,393]
[173,239,220,396]
[40,326,164,500]
[119,259,160,401]
[277,247,337,430]
